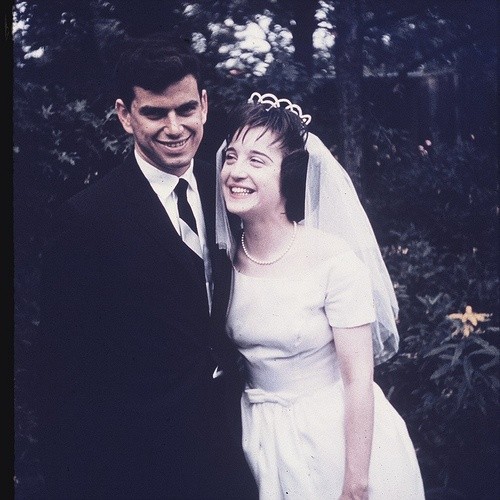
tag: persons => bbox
[53,35,259,500]
[215,92,425,500]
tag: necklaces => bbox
[241,221,297,265]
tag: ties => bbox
[173,179,212,316]
